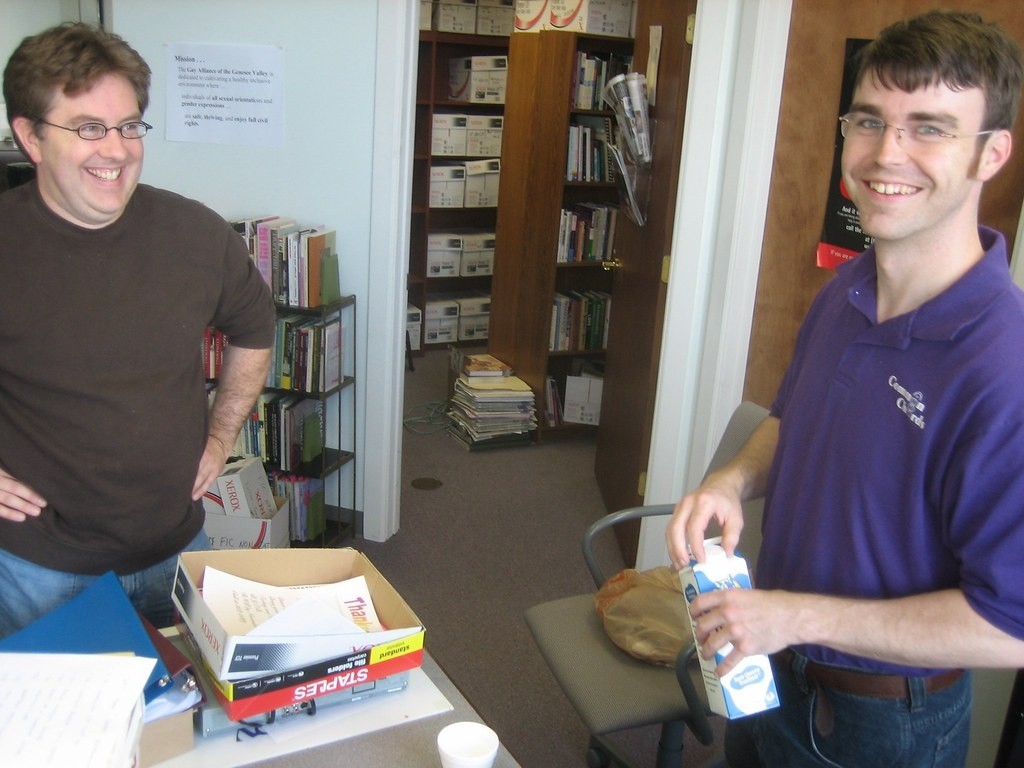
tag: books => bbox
[565,118,615,181]
[557,201,619,263]
[201,215,340,544]
[464,355,512,377]
[572,51,630,111]
[546,376,564,427]
[548,290,611,351]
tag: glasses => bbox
[839,112,993,148]
[35,119,153,140]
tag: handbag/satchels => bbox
[597,566,699,661]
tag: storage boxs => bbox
[404,0,637,355]
[197,458,290,549]
[171,547,426,721]
[132,707,194,768]
[563,363,602,427]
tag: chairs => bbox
[522,401,789,768]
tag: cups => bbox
[437,721,499,768]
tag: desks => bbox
[154,623,522,768]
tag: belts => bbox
[777,649,964,738]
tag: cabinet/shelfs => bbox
[484,30,636,448]
[405,30,511,354]
[203,294,364,555]
[592,0,697,570]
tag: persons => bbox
[0,22,277,642]
[665,8,1024,768]
